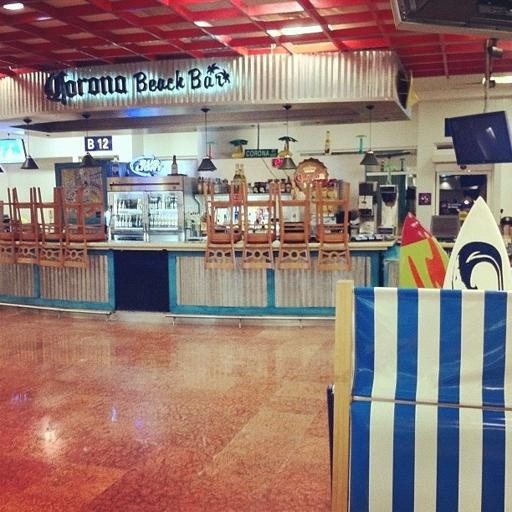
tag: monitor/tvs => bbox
[0,138,26,164]
[430,215,459,243]
[448,110,512,165]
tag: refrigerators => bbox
[103,174,197,246]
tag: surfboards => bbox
[441,197,510,291]
[397,212,449,288]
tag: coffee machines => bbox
[357,171,416,241]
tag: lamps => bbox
[360,105,380,165]
[20,119,38,169]
[81,113,96,162]
[277,105,296,169]
[197,108,217,171]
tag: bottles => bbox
[247,175,292,194]
[197,176,233,194]
[171,154,179,175]
[233,163,246,193]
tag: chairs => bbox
[198,179,351,271]
[1,184,108,269]
